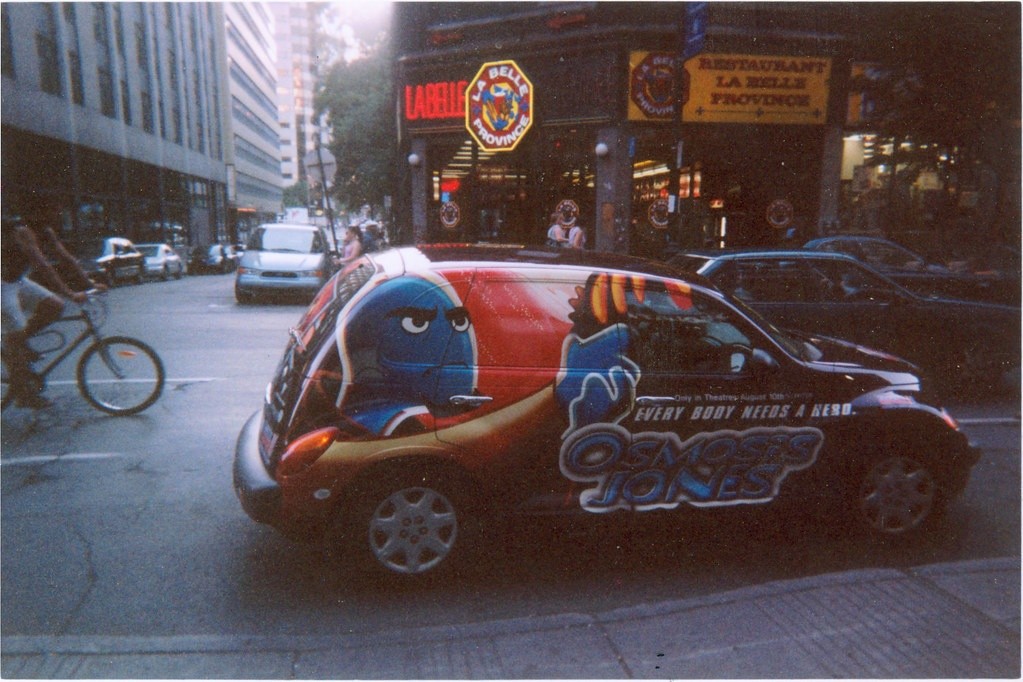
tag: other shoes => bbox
[16,395,56,407]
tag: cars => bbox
[797,235,1022,306]
[190,245,233,275]
[39,237,146,289]
[222,243,240,268]
[234,224,333,305]
[655,249,1022,408]
[136,243,186,281]
[232,240,982,581]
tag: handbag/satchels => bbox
[546,238,559,248]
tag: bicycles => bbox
[0,290,166,419]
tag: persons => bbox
[546,211,590,250]
[332,224,385,272]
[1,188,109,408]
